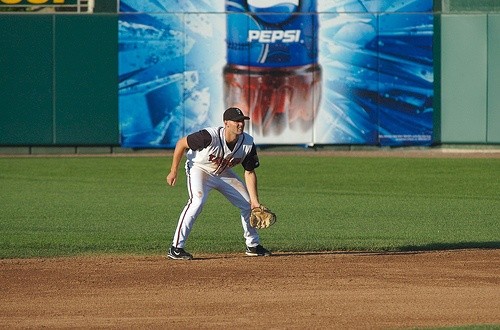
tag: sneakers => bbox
[245,245,271,257]
[167,247,193,261]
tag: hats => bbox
[223,107,250,122]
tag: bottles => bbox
[222,0,322,140]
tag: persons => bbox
[166,108,277,260]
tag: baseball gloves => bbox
[249,205,278,229]
